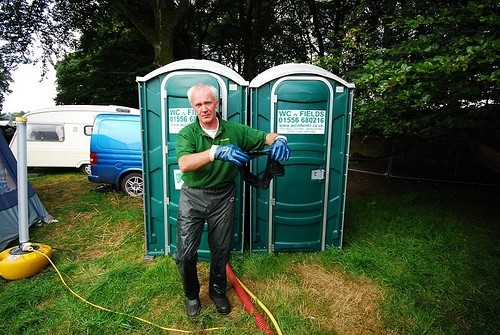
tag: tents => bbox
[0,128,50,252]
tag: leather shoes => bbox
[187,297,201,316]
[209,291,231,314]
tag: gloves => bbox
[265,136,289,160]
[210,144,250,165]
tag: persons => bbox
[176,83,290,318]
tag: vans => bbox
[88,113,143,197]
[8,104,139,175]
